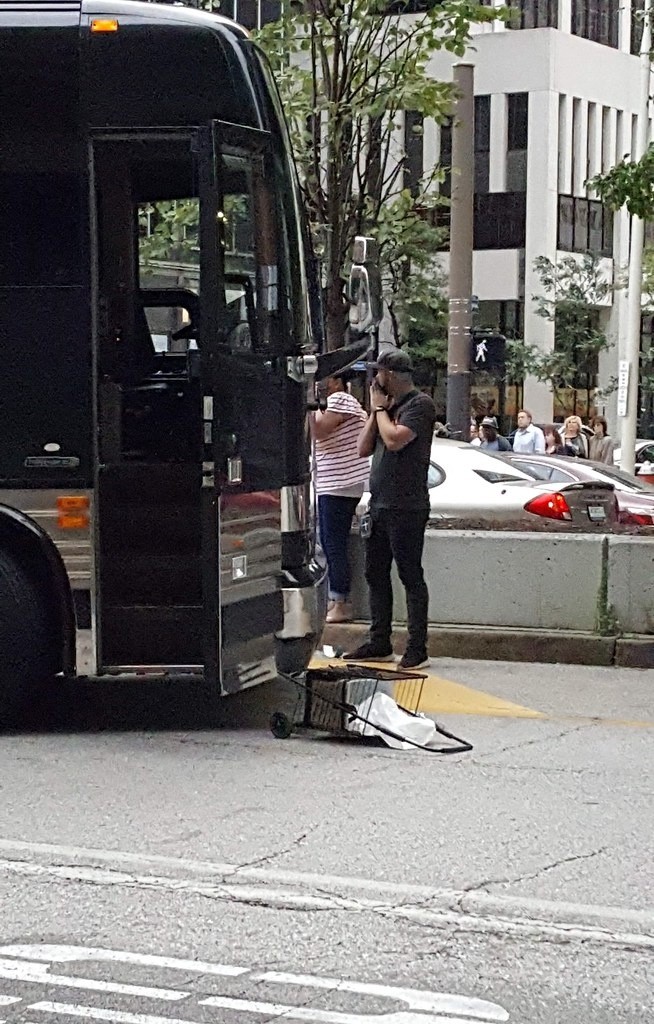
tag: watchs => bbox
[374,406,387,417]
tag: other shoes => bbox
[324,600,352,622]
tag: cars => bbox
[311,424,654,534]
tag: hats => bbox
[479,416,498,428]
[364,348,416,373]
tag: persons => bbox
[342,347,437,670]
[310,374,369,623]
[470,410,614,465]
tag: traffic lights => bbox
[469,333,508,370]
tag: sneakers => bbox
[343,642,395,662]
[396,645,431,670]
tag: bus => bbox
[0,0,383,735]
